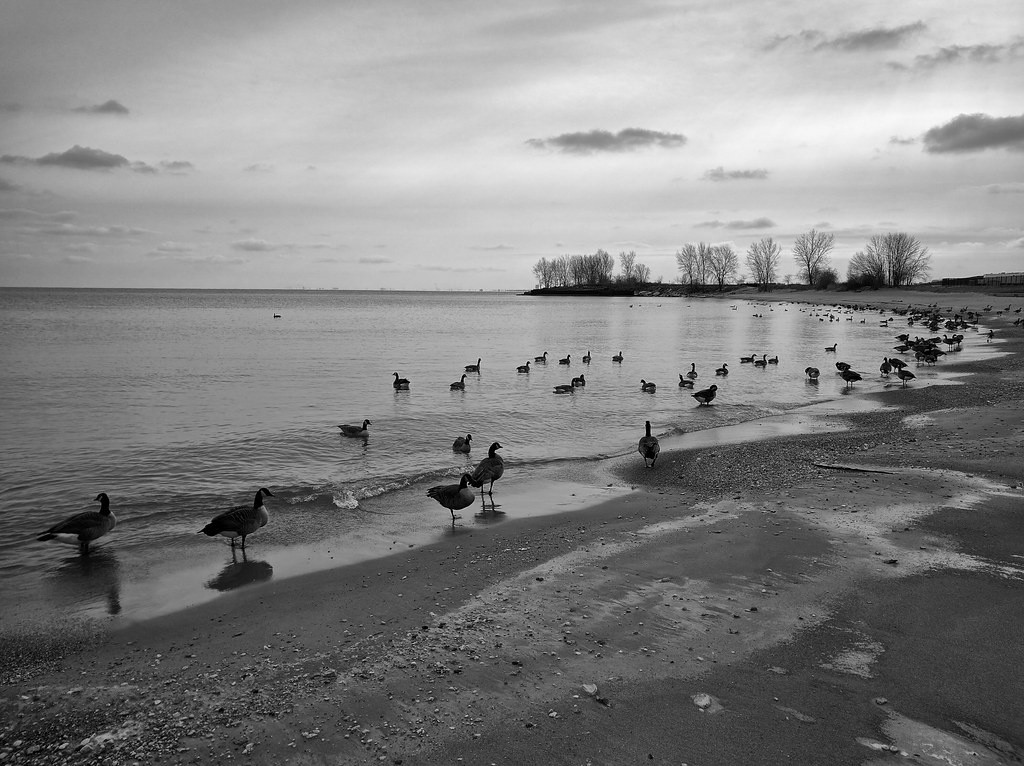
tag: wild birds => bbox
[197,488,275,550]
[464,358,481,372]
[468,442,504,494]
[552,378,577,395]
[273,313,282,319]
[558,355,571,365]
[638,421,660,467]
[393,372,410,390]
[582,351,591,364]
[453,434,473,453]
[427,474,475,519]
[337,419,372,437]
[534,351,548,362]
[36,492,117,550]
[516,361,531,373]
[450,374,467,389]
[629,301,1024,405]
[611,350,623,363]
[574,374,585,387]
